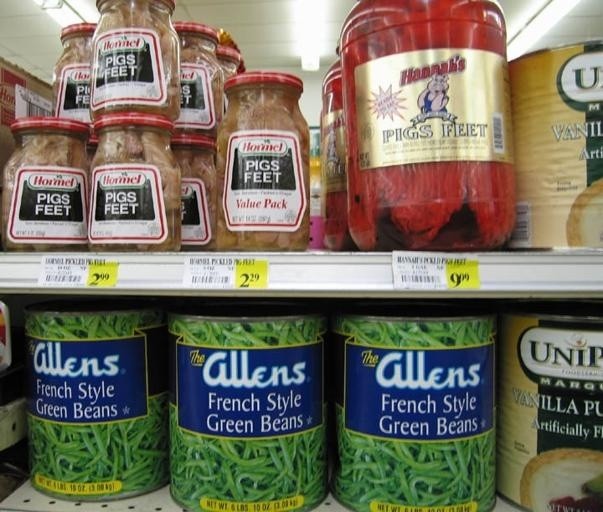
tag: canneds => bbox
[22,306,170,503]
[498,311,603,512]
[504,39,603,253]
[330,311,498,511]
[1,1,310,250]
[165,313,331,511]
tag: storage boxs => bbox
[0,54,58,131]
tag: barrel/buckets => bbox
[323,1,517,249]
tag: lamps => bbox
[505,0,583,60]
[40,0,83,29]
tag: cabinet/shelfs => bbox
[0,242,601,512]
